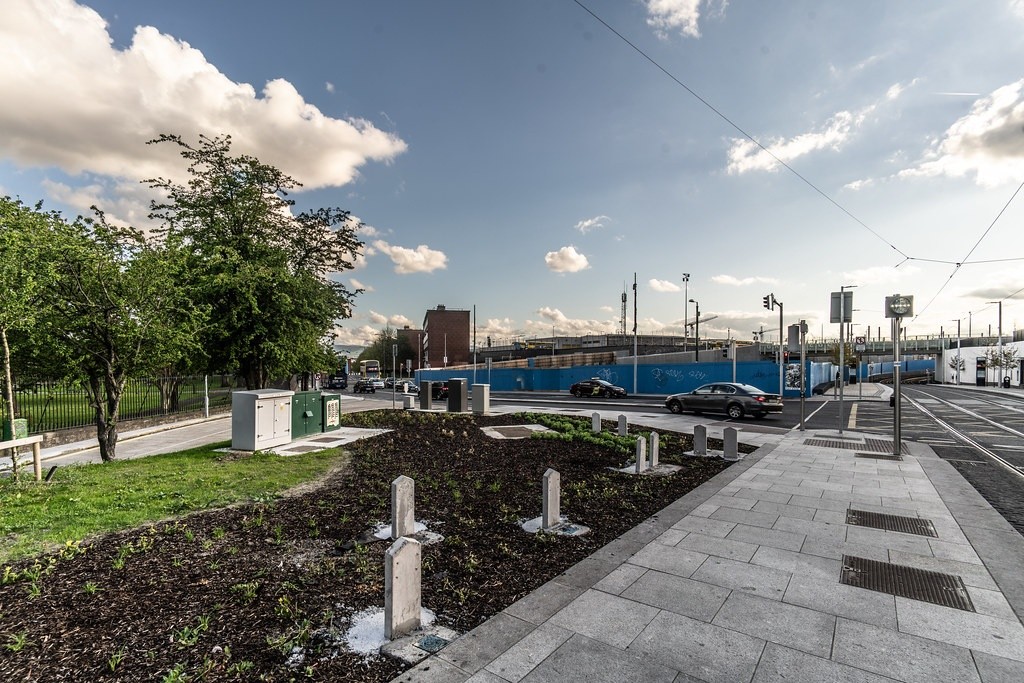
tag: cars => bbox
[369,378,385,389]
[570,379,627,398]
[665,382,784,419]
[395,380,418,393]
[384,376,398,388]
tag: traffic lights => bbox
[763,295,770,309]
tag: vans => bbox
[354,380,376,393]
[418,380,451,400]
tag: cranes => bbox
[752,326,781,342]
[686,315,719,338]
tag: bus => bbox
[320,358,348,389]
[359,360,382,380]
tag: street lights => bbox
[682,273,690,351]
[689,298,700,361]
